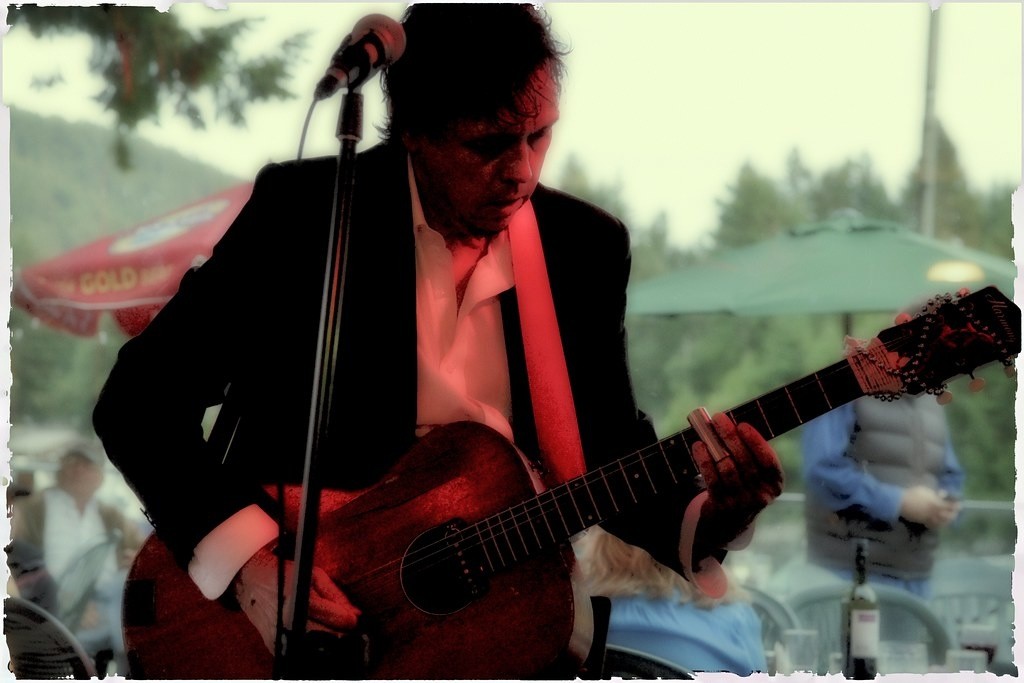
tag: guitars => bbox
[120,282,1021,680]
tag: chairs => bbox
[3,595,95,681]
[741,584,801,676]
[761,583,951,673]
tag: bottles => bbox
[842,545,880,679]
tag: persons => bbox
[798,291,964,603]
[92,3,785,680]
[6,444,146,680]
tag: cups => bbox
[947,650,989,674]
[960,624,998,667]
[882,641,928,674]
[782,629,819,674]
[828,651,842,674]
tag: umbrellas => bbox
[11,181,256,340]
[625,208,1019,360]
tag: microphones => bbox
[314,13,406,101]
